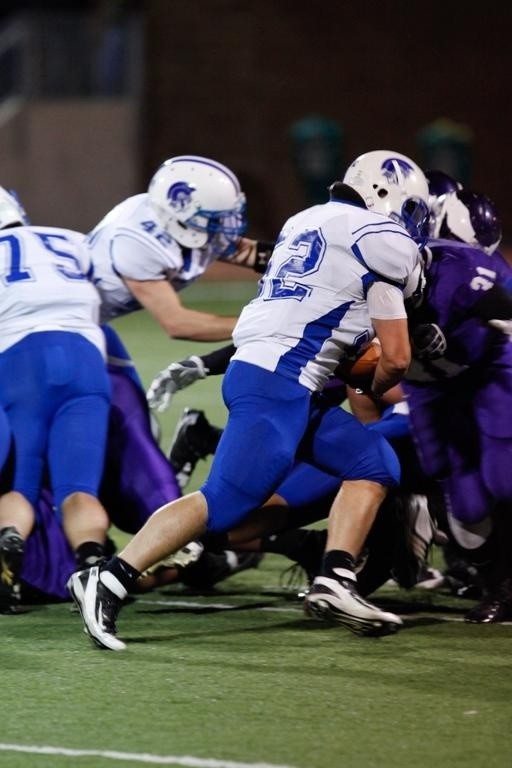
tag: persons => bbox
[1,150,512,652]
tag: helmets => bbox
[145,155,249,256]
[329,149,433,251]
[425,170,463,219]
[432,188,504,257]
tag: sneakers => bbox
[0,529,27,615]
[301,491,512,639]
[65,560,127,651]
[167,407,209,488]
[136,540,204,592]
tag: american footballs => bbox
[351,342,382,376]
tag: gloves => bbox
[411,320,449,362]
[146,355,207,414]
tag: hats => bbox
[0,185,25,229]
